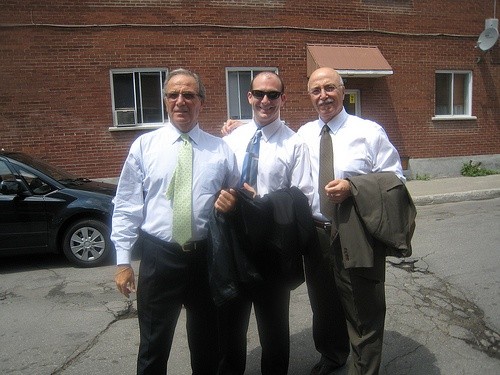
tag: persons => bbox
[221,71,315,375]
[221,67,407,375]
[110,69,240,375]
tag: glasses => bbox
[309,84,343,94]
[249,90,284,100]
[164,92,202,99]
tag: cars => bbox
[0,149,148,268]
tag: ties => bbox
[164,133,193,246]
[239,129,262,193]
[319,125,336,220]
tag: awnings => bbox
[306,44,393,77]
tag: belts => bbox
[312,219,335,232]
[140,231,211,254]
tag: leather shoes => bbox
[310,357,346,375]
